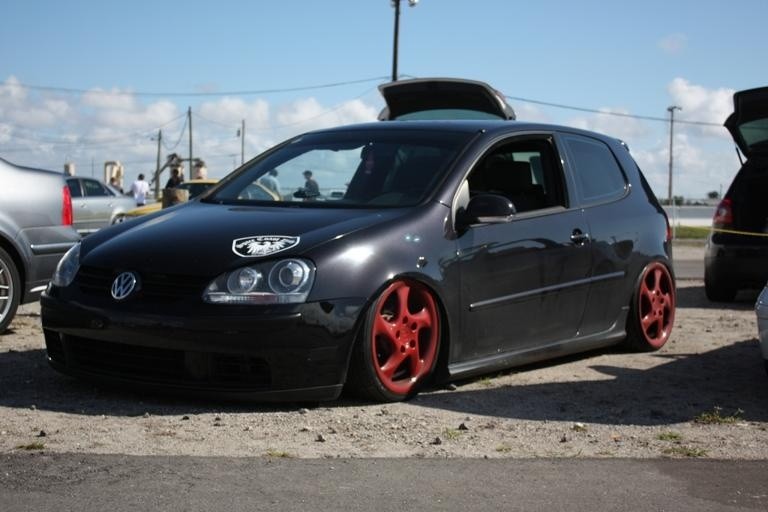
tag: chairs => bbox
[382,155,447,203]
[472,160,543,217]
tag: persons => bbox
[260,168,283,202]
[109,177,124,193]
[299,171,320,201]
[130,173,149,206]
[164,169,183,188]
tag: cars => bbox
[704,85,768,303]
[0,156,81,336]
[65,176,137,239]
[39,78,675,403]
[127,179,281,221]
